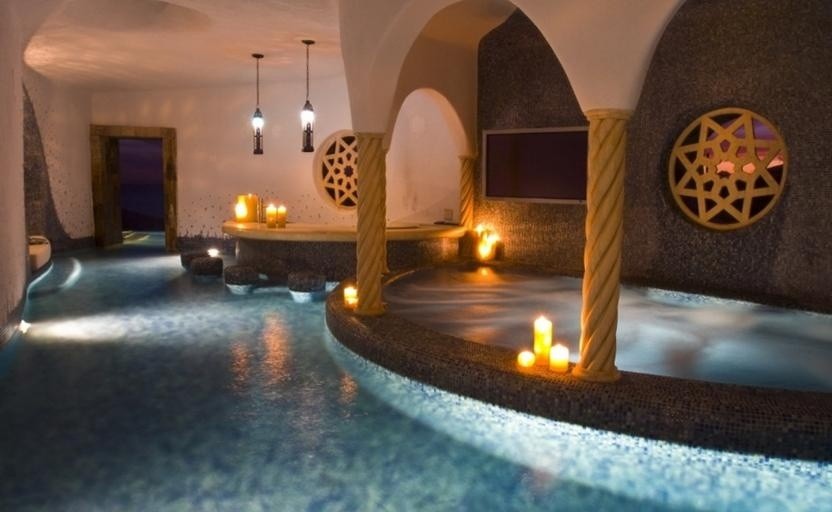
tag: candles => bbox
[277,205,286,229]
[518,352,534,367]
[343,284,357,310]
[534,319,552,366]
[549,344,570,373]
[266,203,276,229]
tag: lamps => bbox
[301,39,315,154]
[250,53,266,155]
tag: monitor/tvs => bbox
[481,126,588,205]
